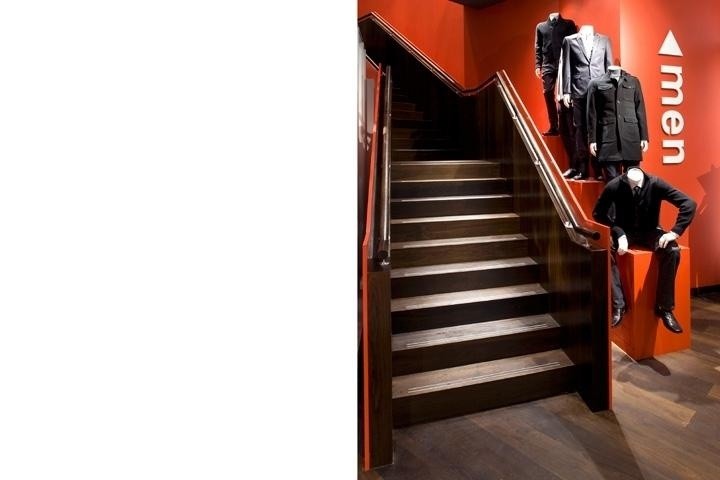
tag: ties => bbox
[634,186,640,202]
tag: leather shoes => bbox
[657,307,682,333]
[598,175,603,180]
[612,307,625,327]
[563,169,577,178]
[543,127,559,136]
[574,172,587,180]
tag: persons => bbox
[535,12,578,136]
[592,166,698,334]
[563,24,613,181]
[586,66,649,183]
[554,48,578,177]
